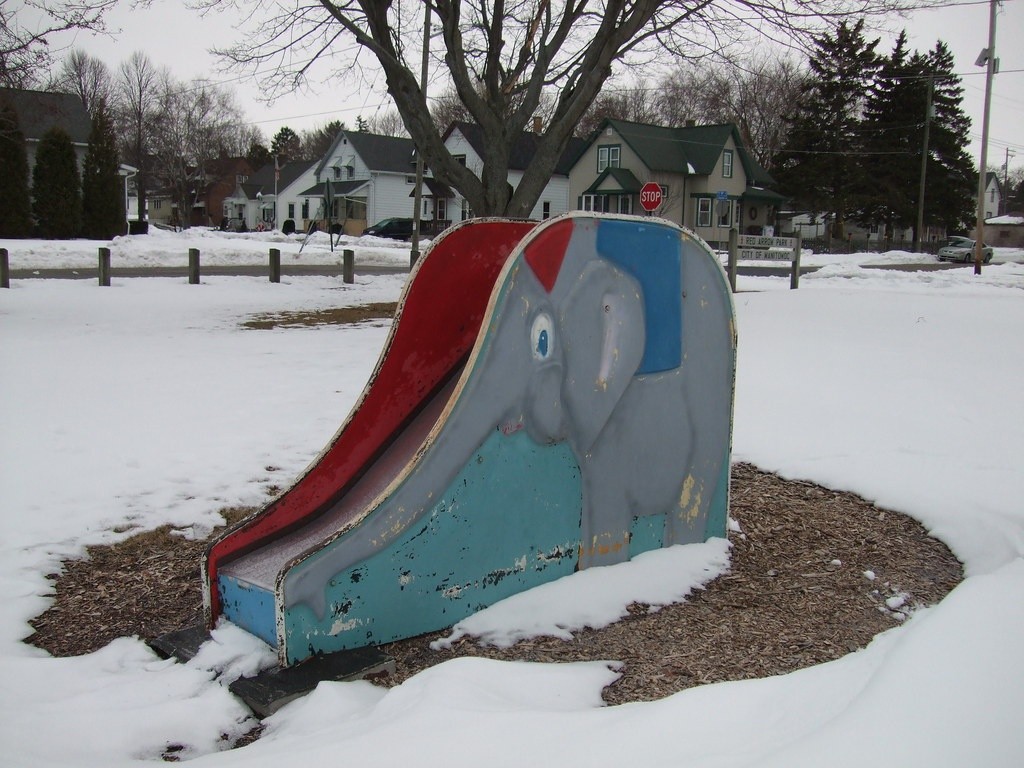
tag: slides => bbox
[202,216,565,595]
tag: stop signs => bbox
[639,182,663,212]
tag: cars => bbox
[360,217,430,241]
[938,240,994,265]
[948,235,972,249]
